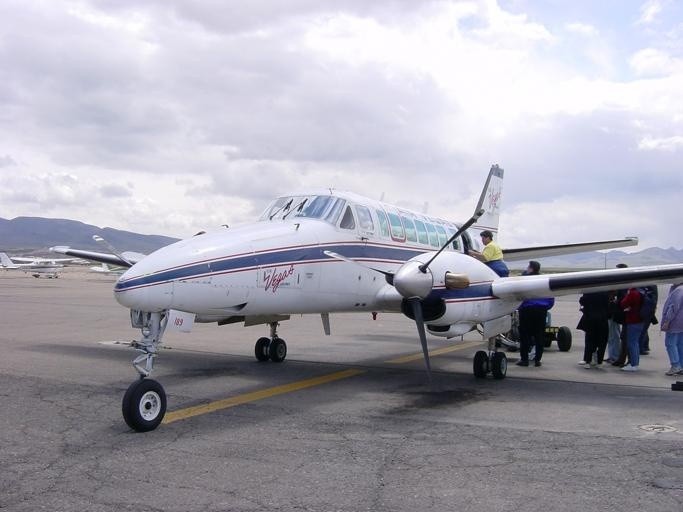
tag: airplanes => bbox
[48,167,683,434]
[0,244,146,281]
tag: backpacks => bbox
[635,287,657,323]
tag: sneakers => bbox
[515,350,542,366]
[577,351,649,371]
[665,365,683,375]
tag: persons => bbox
[575,263,658,372]
[467,230,509,279]
[659,282,682,377]
[516,261,554,368]
